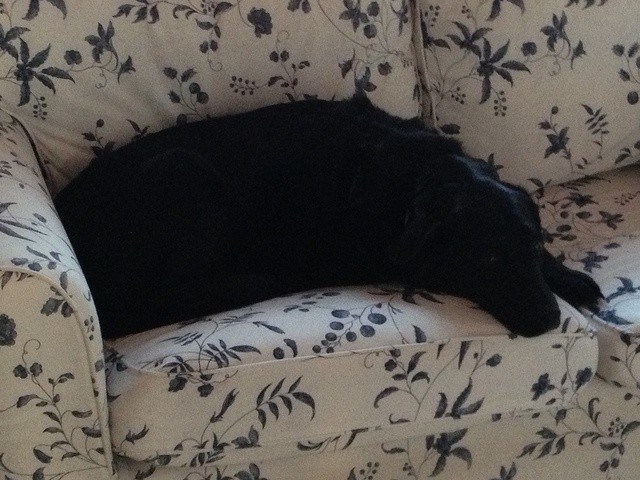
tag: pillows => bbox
[413,3,638,192]
[1,3,421,186]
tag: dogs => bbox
[53,97,604,339]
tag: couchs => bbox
[2,3,640,480]
